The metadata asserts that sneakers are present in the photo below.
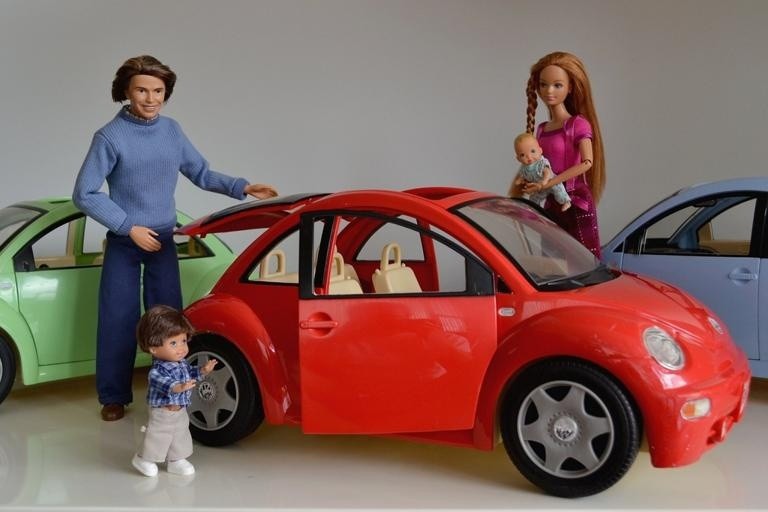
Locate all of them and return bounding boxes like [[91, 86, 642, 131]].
[[100, 403, 125, 422], [128, 453, 197, 478]]
